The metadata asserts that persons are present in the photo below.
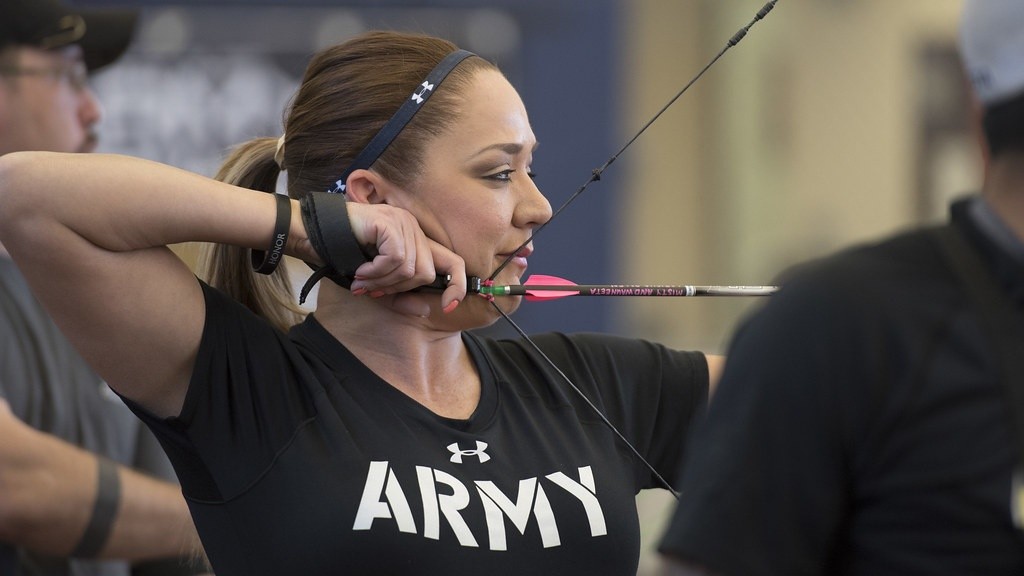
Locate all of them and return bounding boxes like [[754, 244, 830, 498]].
[[650, 1, 1024, 576], [0, 31, 722, 576], [1, 0, 219, 576]]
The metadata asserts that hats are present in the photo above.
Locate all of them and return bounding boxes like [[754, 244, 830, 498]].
[[956, 0, 1023, 106]]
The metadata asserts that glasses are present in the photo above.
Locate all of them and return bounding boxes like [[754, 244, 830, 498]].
[[2, 44, 90, 119]]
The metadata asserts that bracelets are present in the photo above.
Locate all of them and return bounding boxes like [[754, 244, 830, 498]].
[[253, 188, 292, 274], [71, 449, 123, 559]]
